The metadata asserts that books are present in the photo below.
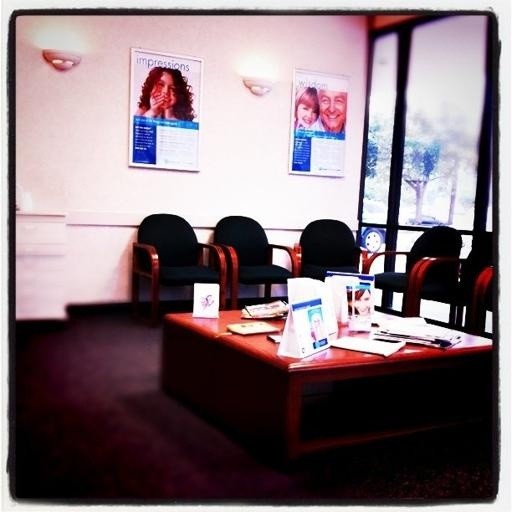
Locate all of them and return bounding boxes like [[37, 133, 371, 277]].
[[331, 336, 406, 358], [241, 300, 289, 319], [373, 324, 463, 350], [226, 321, 279, 334]]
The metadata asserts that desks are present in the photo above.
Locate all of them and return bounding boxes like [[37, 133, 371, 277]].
[[159, 300, 492, 471]]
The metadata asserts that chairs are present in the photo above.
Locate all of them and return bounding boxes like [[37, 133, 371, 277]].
[[409, 230, 493, 337], [291, 218, 369, 283], [211, 215, 297, 311], [363, 221, 457, 328], [129, 211, 228, 323]]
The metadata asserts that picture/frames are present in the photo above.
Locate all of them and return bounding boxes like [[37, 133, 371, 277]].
[[128, 47, 202, 174], [288, 66, 352, 179]]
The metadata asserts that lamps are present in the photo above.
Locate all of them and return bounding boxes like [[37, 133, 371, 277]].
[[42, 47, 82, 72], [242, 74, 277, 97]]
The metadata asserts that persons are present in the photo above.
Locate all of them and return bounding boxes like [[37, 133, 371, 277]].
[[205, 294, 214, 308], [355, 288, 371, 315], [311, 312, 323, 338], [310, 89, 347, 132], [295, 86, 321, 130], [135, 67, 194, 121]]
